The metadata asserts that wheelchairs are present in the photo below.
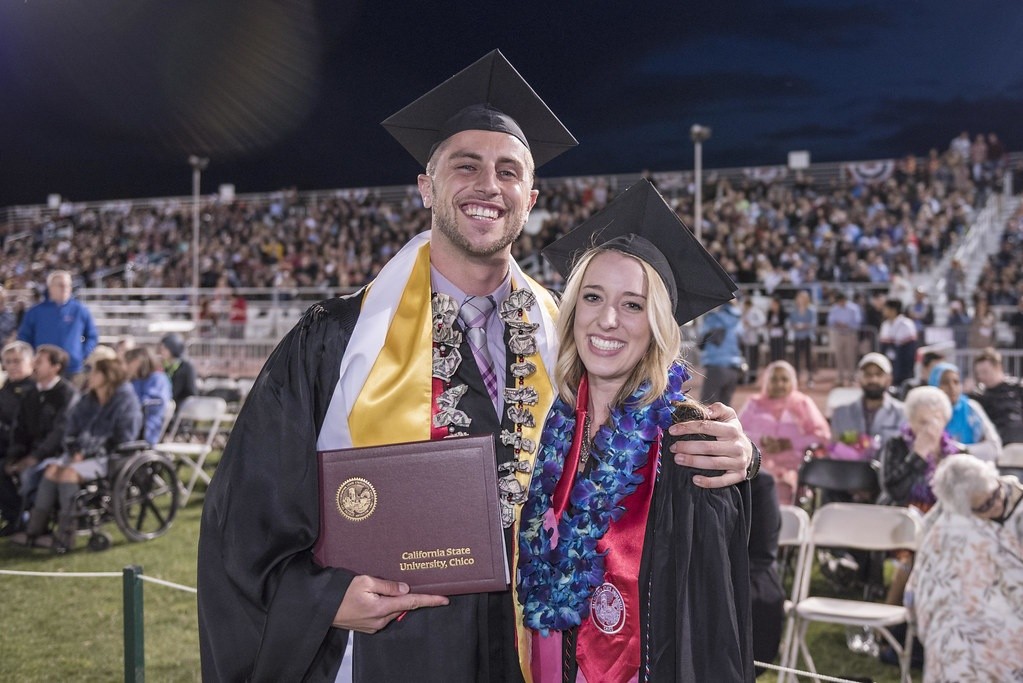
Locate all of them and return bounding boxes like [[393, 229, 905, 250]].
[[7, 440, 187, 557]]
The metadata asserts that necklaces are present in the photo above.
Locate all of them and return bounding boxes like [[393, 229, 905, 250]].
[[515, 358, 692, 638], [579, 416, 592, 463], [431, 281, 540, 529]]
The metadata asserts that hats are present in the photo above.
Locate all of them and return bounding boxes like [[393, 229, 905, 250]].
[[858, 352, 893, 377], [84, 345, 117, 371], [544, 175, 742, 324], [381, 48, 583, 169]]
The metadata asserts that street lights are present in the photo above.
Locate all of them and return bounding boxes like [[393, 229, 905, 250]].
[[691, 122, 714, 243], [188, 155, 209, 337]]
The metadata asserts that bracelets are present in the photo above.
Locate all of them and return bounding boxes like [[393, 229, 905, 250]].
[[746, 443, 761, 480]]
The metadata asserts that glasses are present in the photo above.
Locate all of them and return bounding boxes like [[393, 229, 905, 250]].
[[971, 479, 1002, 514]]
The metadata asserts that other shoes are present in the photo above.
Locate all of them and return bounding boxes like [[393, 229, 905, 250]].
[[52, 523, 76, 556], [11, 525, 47, 545]]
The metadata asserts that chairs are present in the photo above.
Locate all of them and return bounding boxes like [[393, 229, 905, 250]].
[[783, 501, 924, 683], [145, 373, 256, 509], [753, 503, 819, 683], [781, 457, 882, 600]]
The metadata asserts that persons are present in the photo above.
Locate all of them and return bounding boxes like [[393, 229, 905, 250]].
[[122, 346, 172, 446], [951, 129, 1009, 168], [13, 344, 143, 550], [0, 341, 81, 537], [228, 192, 432, 255], [515, 177, 756, 683], [15, 269, 99, 384], [511, 169, 696, 287], [0, 288, 23, 354], [702, 148, 1023, 329], [827, 294, 861, 386], [0, 208, 396, 301], [737, 347, 1023, 643], [878, 299, 918, 399], [198, 49, 759, 683], [696, 298, 746, 405], [766, 288, 815, 389], [159, 332, 197, 432]]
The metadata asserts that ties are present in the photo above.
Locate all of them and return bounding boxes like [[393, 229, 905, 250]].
[[459, 294, 500, 415]]
